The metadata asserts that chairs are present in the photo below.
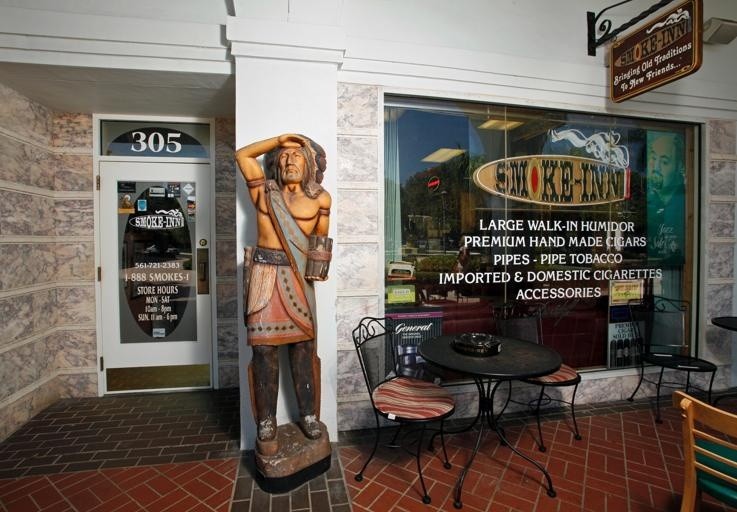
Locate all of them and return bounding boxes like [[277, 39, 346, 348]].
[[352, 313, 451, 504], [624, 291, 737, 511], [493, 297, 584, 454]]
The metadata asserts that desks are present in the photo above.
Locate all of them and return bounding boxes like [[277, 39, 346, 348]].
[[412, 331, 563, 510]]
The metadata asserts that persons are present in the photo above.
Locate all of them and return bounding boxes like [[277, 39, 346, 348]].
[[234, 134, 332, 442], [646, 137, 684, 257]]
